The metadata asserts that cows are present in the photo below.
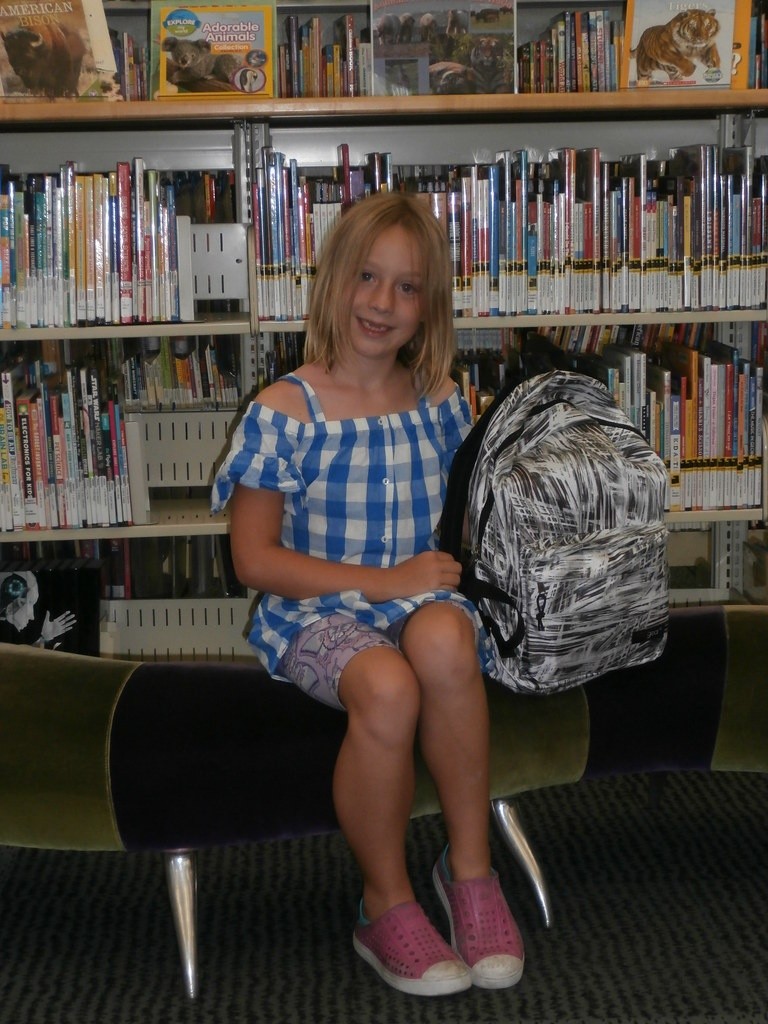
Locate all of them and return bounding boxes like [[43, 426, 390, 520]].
[[471, 6, 513, 22]]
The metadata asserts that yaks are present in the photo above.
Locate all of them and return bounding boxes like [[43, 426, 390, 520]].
[[0, 18, 89, 98]]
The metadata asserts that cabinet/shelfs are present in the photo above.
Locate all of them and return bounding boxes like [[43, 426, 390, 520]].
[[0, 0, 768, 662]]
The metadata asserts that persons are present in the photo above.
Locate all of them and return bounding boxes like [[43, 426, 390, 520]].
[[208, 192, 524, 994]]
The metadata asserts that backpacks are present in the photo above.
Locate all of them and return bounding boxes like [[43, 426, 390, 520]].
[[439, 334, 670, 693]]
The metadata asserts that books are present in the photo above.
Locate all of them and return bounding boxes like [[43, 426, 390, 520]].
[[109, 27, 151, 101], [0, 144, 768, 329], [452, 322, 768, 534], [518, 10, 625, 94], [278, 14, 371, 98], [0, 333, 308, 598], [749, 14, 768, 89]]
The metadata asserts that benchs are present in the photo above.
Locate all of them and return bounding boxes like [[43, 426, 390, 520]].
[[0, 604, 768, 998]]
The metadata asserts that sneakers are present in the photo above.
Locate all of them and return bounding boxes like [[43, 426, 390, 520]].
[[354, 901, 472, 996], [432, 842, 525, 990]]
